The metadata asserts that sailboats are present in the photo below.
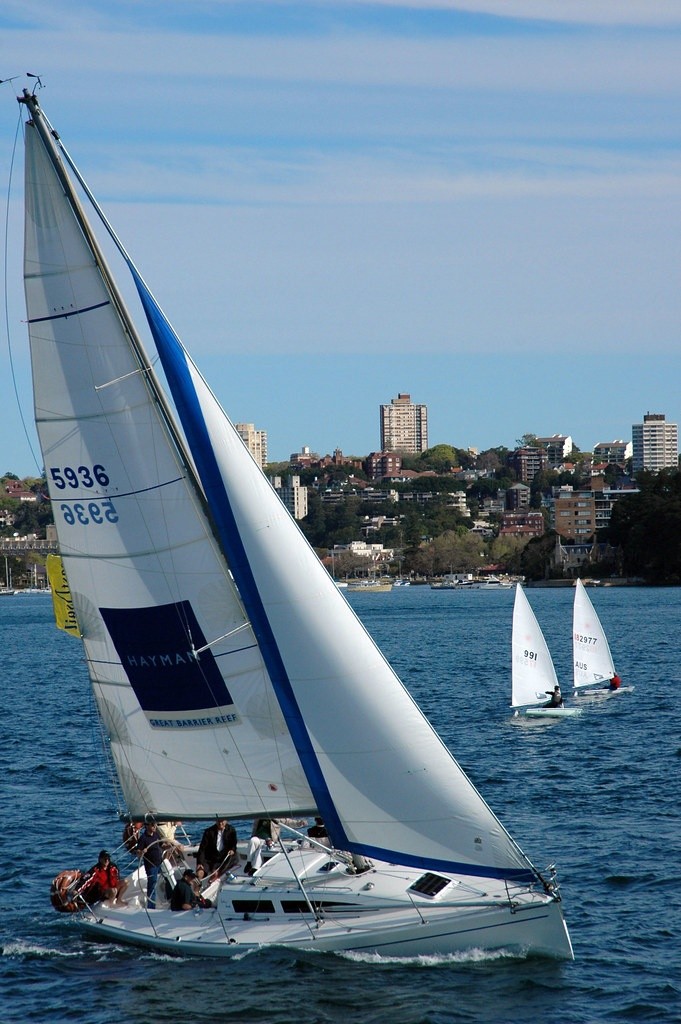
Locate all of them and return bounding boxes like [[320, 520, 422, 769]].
[[571, 577, 636, 696], [508, 582, 584, 718], [4, 66, 579, 961]]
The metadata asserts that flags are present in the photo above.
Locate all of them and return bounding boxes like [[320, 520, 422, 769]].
[[45, 553, 81, 639]]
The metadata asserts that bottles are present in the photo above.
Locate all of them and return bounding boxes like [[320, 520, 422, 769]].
[[194, 904, 200, 916], [226, 870, 234, 879]]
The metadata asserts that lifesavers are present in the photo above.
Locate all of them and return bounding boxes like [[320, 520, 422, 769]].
[[123, 822, 145, 856], [50, 870, 90, 914]]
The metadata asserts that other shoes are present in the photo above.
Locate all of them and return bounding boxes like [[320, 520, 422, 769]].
[[244, 862, 252, 872], [248, 868, 256, 876]]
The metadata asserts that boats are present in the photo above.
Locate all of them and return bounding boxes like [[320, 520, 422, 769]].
[[347, 576, 393, 593], [429, 563, 514, 590], [583, 578, 602, 588], [392, 579, 410, 588]]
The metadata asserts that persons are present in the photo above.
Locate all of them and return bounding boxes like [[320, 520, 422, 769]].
[[302, 817, 332, 849], [603, 672, 621, 691], [91, 850, 132, 908], [541, 686, 563, 708], [123, 817, 237, 912], [244, 817, 309, 877]]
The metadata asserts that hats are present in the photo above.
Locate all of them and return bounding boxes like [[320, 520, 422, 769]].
[[100, 850, 110, 857], [184, 869, 196, 879]]
